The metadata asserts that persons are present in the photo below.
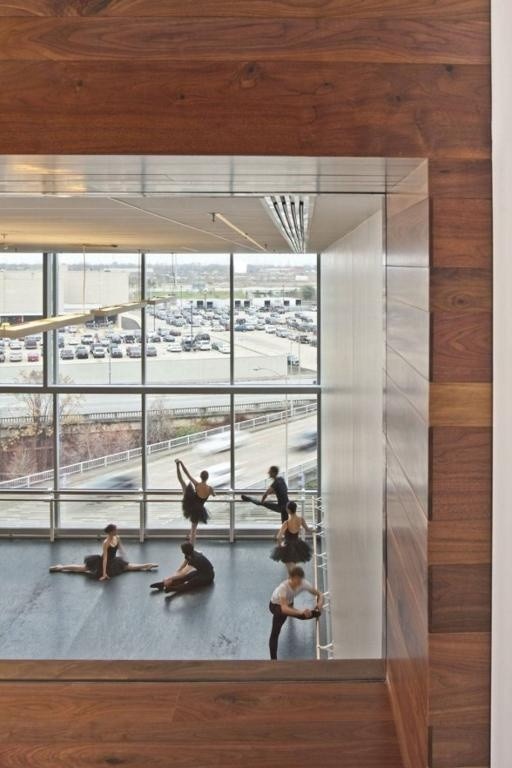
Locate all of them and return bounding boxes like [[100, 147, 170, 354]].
[[268, 567, 322, 659], [242, 466, 288, 523], [269, 501, 314, 574], [175, 457, 216, 545], [48, 524, 160, 581], [150, 542, 215, 592]]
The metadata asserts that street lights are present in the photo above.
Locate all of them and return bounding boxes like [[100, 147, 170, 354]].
[[105, 319, 117, 385], [251, 368, 288, 490], [180, 285, 183, 308]]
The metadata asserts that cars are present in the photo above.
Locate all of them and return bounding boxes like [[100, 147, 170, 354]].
[[184, 460, 249, 493], [289, 426, 318, 456], [194, 429, 251, 457], [0, 298, 317, 362], [84, 471, 142, 506]]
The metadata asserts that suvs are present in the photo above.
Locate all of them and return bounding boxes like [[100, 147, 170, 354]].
[[286, 356, 300, 366]]
